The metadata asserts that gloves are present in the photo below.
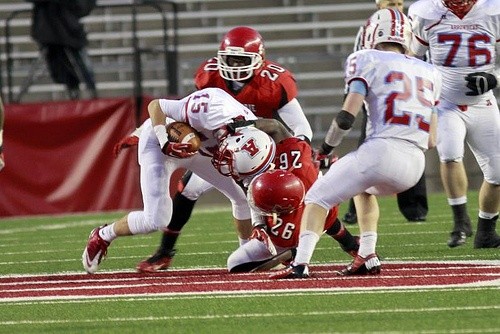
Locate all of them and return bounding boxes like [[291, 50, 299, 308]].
[[161, 140, 199, 159], [114, 135, 139, 159], [315, 151, 338, 170], [249, 224, 268, 241], [464, 72, 497, 96]]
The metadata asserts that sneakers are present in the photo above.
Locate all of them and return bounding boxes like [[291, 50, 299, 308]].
[[268, 261, 309, 279], [341, 236, 380, 261], [474, 231, 500, 249], [136, 256, 172, 273], [82, 224, 110, 276], [282, 248, 296, 267], [448, 217, 473, 247], [337, 254, 381, 277]]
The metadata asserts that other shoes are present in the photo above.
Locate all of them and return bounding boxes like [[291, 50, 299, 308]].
[[342, 212, 357, 224]]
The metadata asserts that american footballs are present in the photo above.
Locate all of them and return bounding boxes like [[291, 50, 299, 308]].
[[165, 121, 201, 153]]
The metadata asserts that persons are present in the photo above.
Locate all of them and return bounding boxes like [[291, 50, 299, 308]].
[[23, 0, 100, 100], [406, 0, 500, 249], [81, 0, 360, 273], [343, 0, 429, 224], [268, 6, 443, 278]]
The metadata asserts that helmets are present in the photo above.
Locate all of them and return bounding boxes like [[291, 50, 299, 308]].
[[211, 124, 276, 179], [218, 26, 264, 81], [360, 8, 413, 53], [247, 169, 305, 216]]
